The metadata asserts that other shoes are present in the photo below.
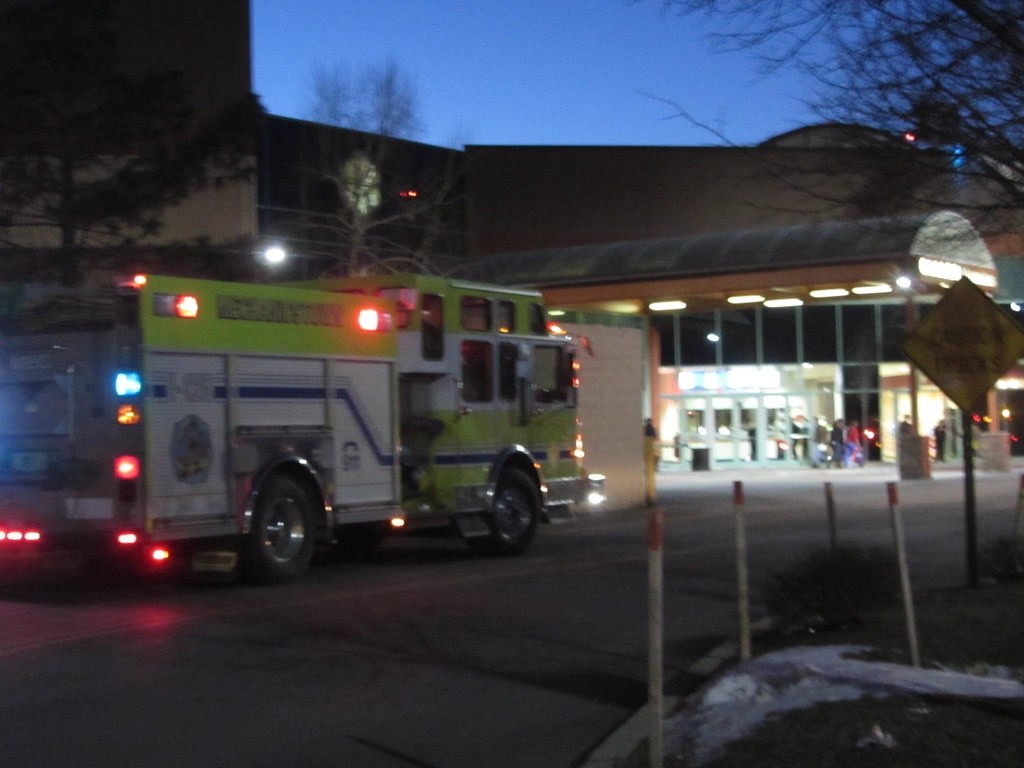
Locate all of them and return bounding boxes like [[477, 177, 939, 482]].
[[834, 461, 843, 468], [936, 456, 945, 461]]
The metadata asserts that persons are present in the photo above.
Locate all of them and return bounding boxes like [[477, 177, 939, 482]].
[[793, 415, 859, 470], [900, 415, 912, 436], [749, 424, 755, 460], [935, 421, 945, 460], [643, 418, 656, 438]]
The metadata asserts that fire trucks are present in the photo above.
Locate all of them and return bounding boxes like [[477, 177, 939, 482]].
[[0, 273, 594, 583]]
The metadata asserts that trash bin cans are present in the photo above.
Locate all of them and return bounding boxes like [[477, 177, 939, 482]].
[[689, 447, 710, 471]]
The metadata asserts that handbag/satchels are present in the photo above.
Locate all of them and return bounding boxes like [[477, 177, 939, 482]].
[[850, 448, 866, 466]]
